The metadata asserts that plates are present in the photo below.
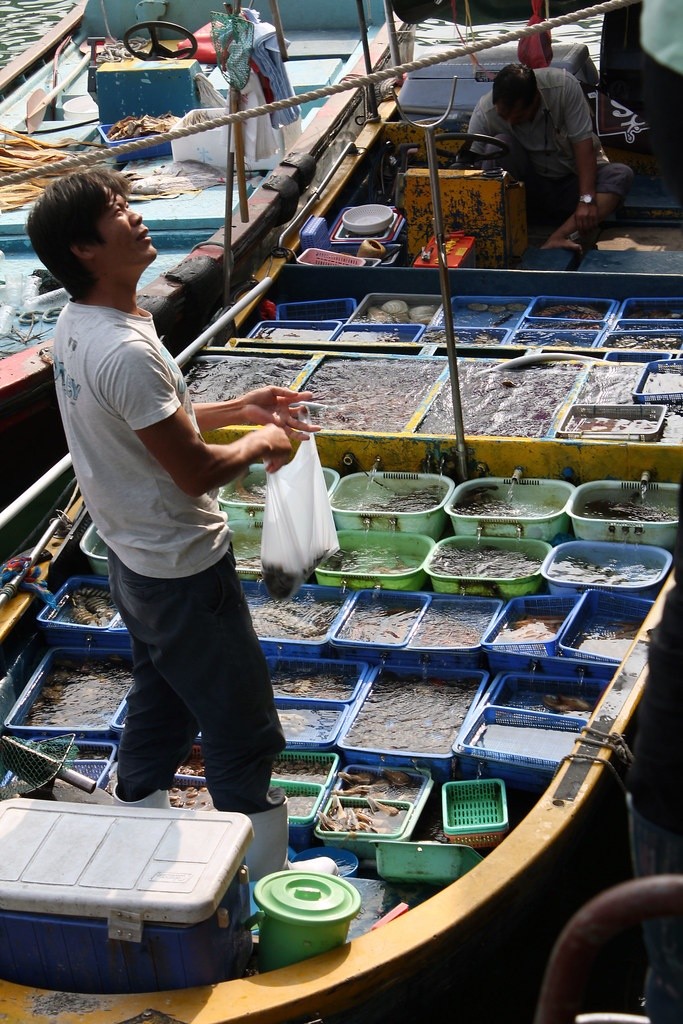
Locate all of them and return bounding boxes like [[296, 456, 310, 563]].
[[290, 847, 359, 879]]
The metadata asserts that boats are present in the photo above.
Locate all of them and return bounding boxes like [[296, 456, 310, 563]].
[[1, 1, 682, 1024], [0, 1, 408, 451]]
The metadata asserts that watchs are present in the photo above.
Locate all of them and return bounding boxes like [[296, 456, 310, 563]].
[[577, 195, 597, 206]]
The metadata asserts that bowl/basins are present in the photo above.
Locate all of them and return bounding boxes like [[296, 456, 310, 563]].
[[341, 204, 394, 235]]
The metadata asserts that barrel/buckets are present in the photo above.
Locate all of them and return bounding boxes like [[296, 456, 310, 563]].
[[245, 870, 361, 970], [62, 95, 101, 122]]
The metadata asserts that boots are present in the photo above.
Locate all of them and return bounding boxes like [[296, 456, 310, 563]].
[[113, 782, 172, 810], [245, 795, 340, 881]]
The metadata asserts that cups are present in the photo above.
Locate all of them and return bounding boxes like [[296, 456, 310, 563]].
[[354, 239, 387, 260]]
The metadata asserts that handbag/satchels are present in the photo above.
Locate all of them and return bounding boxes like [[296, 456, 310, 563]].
[[259, 405, 342, 601]]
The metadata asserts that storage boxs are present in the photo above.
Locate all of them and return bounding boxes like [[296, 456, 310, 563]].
[[0, 201, 683, 997]]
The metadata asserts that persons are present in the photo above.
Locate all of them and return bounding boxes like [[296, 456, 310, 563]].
[[28, 165, 346, 887], [466, 63, 636, 257]]
